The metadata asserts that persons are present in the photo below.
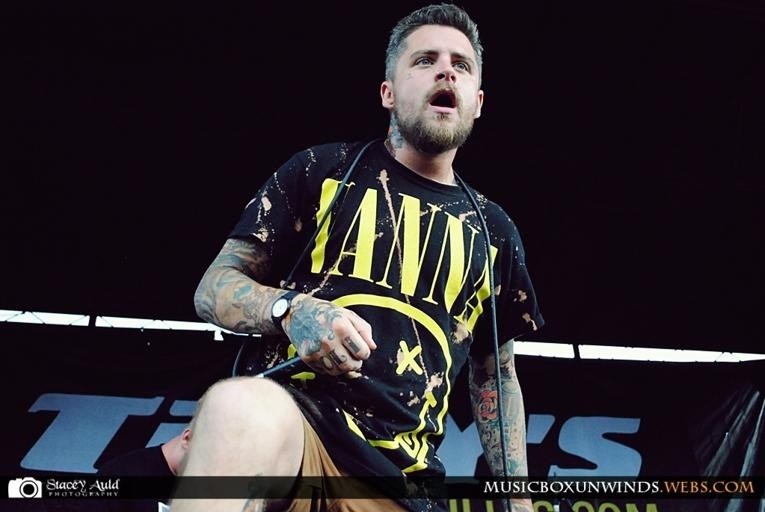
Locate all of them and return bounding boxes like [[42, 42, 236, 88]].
[[91, 413, 203, 512], [170, 0, 548, 512]]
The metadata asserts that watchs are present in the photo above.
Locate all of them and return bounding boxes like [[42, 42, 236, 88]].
[[269, 288, 303, 336]]
